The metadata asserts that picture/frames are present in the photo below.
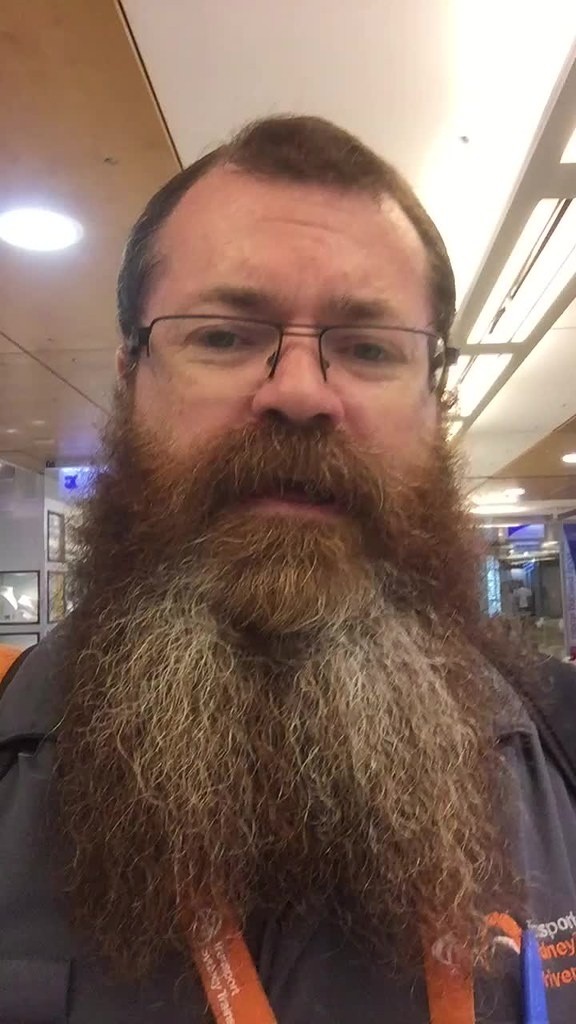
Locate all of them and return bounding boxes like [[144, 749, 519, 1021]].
[[46, 570, 66, 623], [1, 570, 41, 626], [0, 632, 40, 650], [47, 511, 66, 563]]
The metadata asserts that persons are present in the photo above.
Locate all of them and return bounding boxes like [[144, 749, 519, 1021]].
[[0, 117, 576, 1024]]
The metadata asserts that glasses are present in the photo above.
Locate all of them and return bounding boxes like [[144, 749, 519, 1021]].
[[128, 316, 461, 413]]
